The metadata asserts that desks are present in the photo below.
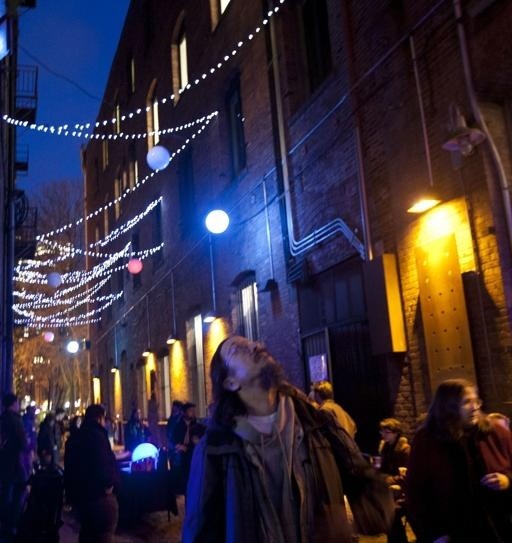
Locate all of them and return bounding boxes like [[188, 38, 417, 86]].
[[118, 473, 185, 522]]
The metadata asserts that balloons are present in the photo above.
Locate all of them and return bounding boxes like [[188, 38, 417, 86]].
[[146, 145, 170, 171], [127, 258, 143, 274], [46, 271, 60, 288]]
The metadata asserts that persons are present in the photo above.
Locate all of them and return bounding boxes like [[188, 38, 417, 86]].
[[1, 336, 511, 543]]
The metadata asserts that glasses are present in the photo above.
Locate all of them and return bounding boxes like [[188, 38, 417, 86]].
[[379, 430, 392, 435], [460, 397, 483, 410]]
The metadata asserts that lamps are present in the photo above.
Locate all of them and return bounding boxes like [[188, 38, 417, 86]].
[[439, 103, 486, 171], [256, 278, 274, 293], [91, 313, 214, 384]]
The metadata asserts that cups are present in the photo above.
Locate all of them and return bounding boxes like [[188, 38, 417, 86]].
[[398, 467, 408, 477], [373, 454, 382, 469]]
